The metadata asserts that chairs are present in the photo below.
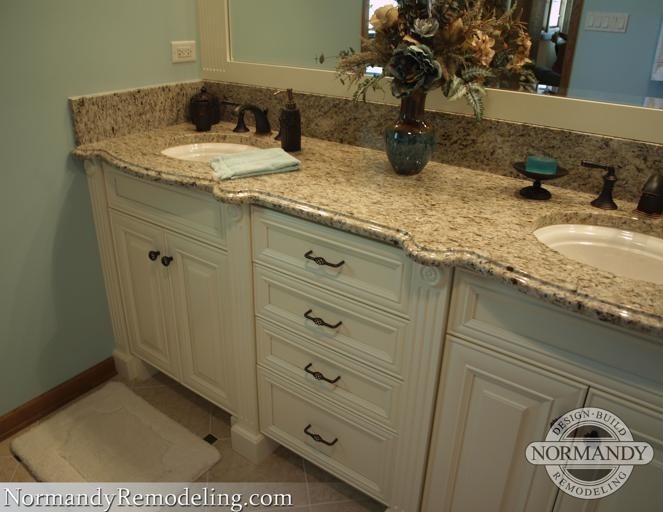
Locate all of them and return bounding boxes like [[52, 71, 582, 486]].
[[532, 30, 567, 87]]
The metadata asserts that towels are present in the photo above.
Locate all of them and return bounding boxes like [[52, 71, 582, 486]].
[[209, 147, 301, 180]]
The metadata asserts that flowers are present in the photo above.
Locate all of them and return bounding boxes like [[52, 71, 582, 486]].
[[314, 0, 510, 129], [484, 2, 537, 94]]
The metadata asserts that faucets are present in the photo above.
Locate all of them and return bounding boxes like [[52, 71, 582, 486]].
[[233, 102, 271, 135], [636, 172, 663, 217]]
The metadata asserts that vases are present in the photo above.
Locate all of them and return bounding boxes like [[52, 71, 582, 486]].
[[384, 91, 433, 176]]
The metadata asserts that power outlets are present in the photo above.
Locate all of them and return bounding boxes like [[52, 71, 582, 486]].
[[171, 40, 195, 63]]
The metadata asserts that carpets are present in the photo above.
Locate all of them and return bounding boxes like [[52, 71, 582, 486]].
[[10, 379, 223, 482]]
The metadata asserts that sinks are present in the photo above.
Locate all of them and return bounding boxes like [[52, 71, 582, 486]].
[[530, 210, 663, 286], [159, 133, 274, 163]]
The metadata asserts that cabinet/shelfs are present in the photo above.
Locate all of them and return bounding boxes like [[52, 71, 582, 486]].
[[246, 201, 454, 512], [418, 266, 663, 512], [83, 157, 261, 464]]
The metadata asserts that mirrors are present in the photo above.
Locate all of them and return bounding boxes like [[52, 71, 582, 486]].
[[194, 1, 663, 144]]
